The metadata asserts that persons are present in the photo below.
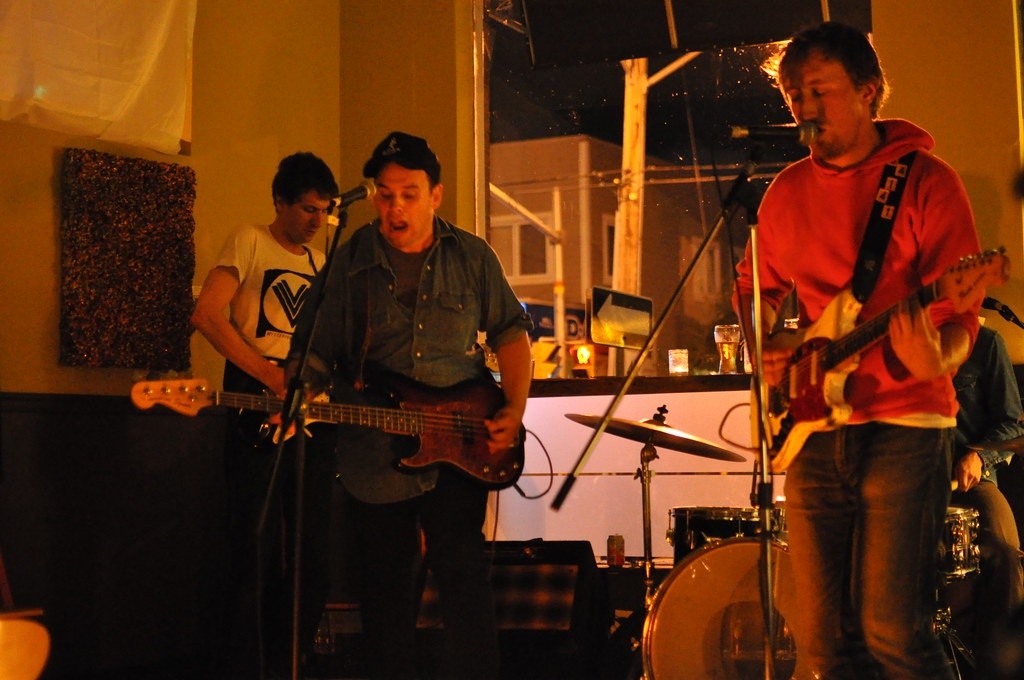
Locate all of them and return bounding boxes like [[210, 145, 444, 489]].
[[283, 132, 530, 680], [193, 149, 338, 680], [948, 326, 1024, 611], [729, 21, 986, 680]]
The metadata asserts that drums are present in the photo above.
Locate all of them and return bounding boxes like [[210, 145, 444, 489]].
[[642, 536, 797, 680], [671, 506, 784, 568], [938, 505, 983, 580]]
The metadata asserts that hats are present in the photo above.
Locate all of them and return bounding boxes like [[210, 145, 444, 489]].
[[363, 131, 440, 178]]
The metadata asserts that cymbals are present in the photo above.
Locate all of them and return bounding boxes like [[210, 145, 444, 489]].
[[564, 412, 749, 464], [967, 434, 1024, 454]]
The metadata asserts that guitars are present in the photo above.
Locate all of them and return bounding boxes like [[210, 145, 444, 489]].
[[764, 245, 1014, 476], [270, 342, 501, 445], [127, 368, 528, 491]]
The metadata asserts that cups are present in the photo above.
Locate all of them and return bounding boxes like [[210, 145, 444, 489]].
[[715, 324, 740, 375]]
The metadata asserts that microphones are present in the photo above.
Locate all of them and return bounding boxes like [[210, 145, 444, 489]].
[[328, 179, 377, 212], [724, 121, 818, 144]]
